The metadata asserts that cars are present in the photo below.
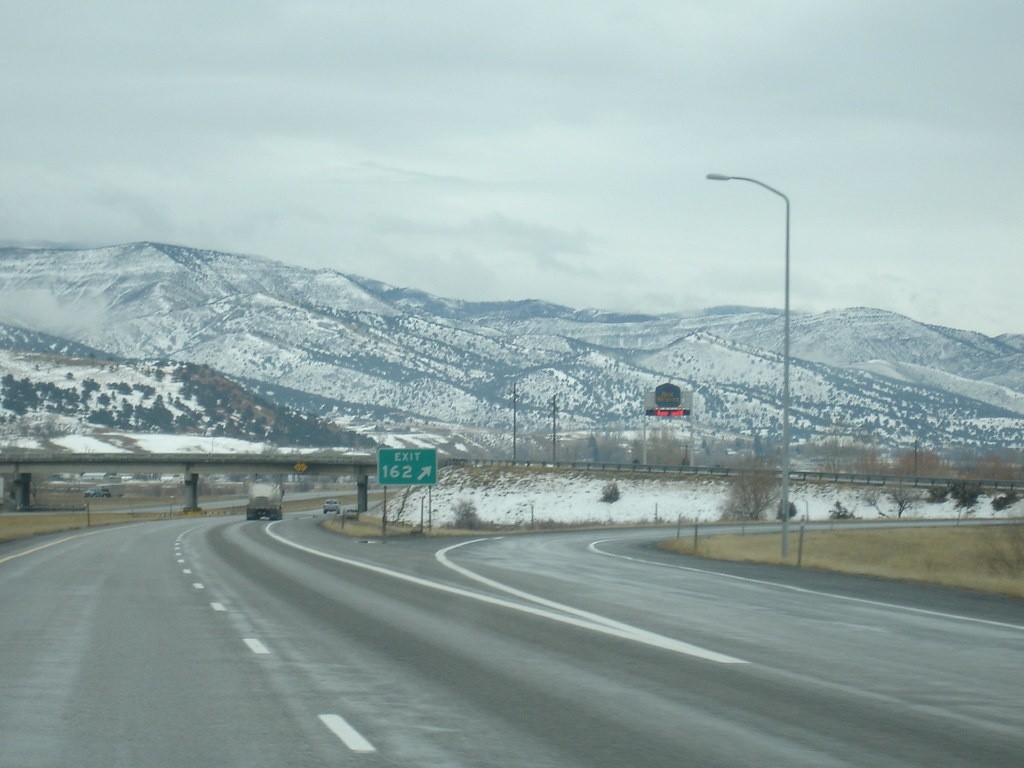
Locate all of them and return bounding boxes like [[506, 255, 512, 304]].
[[323, 499, 341, 514], [83, 486, 111, 499]]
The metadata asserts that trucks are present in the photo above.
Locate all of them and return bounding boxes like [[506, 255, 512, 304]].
[[245, 474, 285, 521]]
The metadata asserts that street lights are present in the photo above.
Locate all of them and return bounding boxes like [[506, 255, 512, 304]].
[[707, 172, 791, 558]]
